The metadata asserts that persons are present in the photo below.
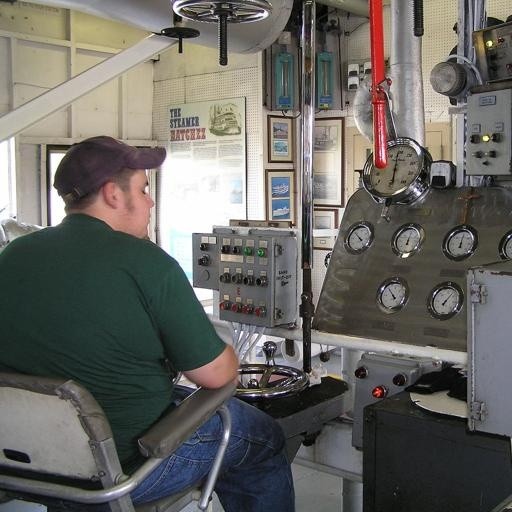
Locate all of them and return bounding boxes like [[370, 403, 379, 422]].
[[0, 136, 295, 510]]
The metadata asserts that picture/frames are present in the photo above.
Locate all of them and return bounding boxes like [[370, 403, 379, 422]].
[[267, 115, 294, 162], [313, 117, 345, 208], [312, 209, 339, 230], [266, 168, 297, 223]]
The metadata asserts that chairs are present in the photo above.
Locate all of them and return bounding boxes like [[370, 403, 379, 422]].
[[0, 370, 241, 512]]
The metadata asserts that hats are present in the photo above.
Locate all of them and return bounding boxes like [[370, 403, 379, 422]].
[[53, 137, 166, 204]]
